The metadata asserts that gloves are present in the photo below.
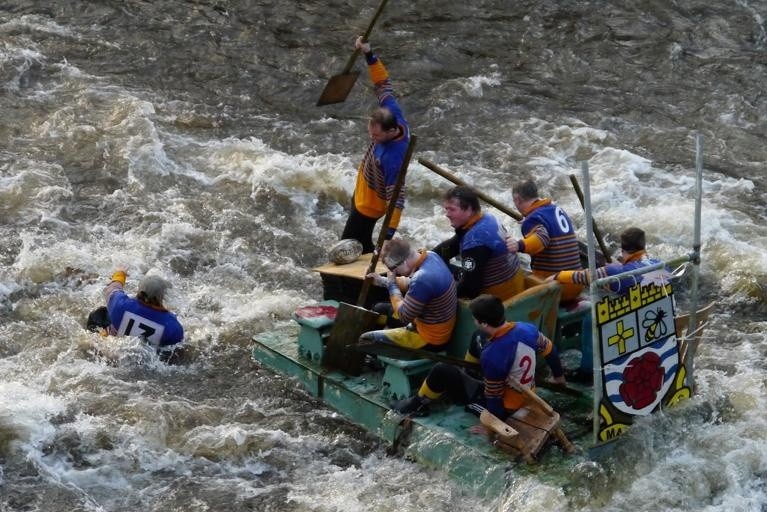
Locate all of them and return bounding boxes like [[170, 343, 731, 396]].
[[362, 267, 381, 286], [387, 271, 397, 290]]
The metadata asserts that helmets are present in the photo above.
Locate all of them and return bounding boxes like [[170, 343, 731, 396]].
[[327, 238, 364, 265]]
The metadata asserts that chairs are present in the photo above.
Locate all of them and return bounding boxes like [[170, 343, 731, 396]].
[[676, 301, 714, 366], [448, 274, 565, 363], [479, 375, 575, 465]]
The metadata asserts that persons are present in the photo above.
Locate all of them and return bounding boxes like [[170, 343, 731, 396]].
[[88, 266, 183, 347], [389, 294, 566, 422], [545, 228, 672, 384], [341, 35, 412, 261], [506, 179, 586, 305], [430, 184, 526, 303], [358, 238, 457, 372]]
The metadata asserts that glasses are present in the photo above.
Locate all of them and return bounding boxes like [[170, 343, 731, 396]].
[[388, 260, 403, 272]]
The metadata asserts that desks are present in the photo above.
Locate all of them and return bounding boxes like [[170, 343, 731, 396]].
[[310, 252, 390, 306]]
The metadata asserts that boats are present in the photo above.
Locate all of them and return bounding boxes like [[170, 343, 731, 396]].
[[248, 131, 734, 512]]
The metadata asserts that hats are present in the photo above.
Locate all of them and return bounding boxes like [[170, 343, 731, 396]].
[[137, 275, 172, 299]]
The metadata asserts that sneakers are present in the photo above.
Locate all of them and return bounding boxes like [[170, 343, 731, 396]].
[[389, 392, 430, 417], [560, 367, 592, 386]]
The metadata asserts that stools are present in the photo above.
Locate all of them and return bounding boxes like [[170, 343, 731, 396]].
[[558, 300, 593, 368], [293, 301, 340, 362], [378, 350, 447, 400]]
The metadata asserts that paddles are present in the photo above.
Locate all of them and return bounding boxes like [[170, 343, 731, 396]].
[[417, 155, 607, 270], [346, 340, 584, 400], [320, 134, 421, 377], [316, 0, 388, 106]]
